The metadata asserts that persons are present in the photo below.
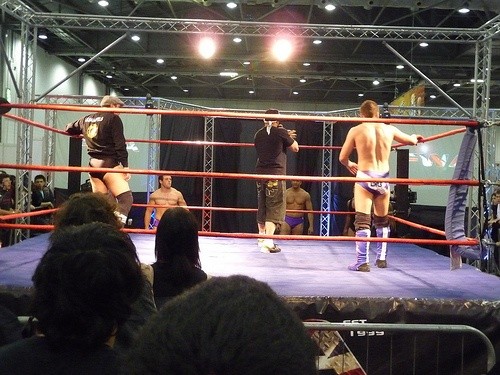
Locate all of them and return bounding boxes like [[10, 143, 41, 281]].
[[275, 179, 314, 235], [343, 196, 397, 236], [0, 171, 319, 375], [65, 95, 133, 228], [253, 108, 299, 253], [339, 99, 424, 272], [144, 175, 189, 230], [490, 189, 500, 277]]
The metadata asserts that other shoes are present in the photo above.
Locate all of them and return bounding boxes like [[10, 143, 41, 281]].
[[348, 262, 370, 271], [261, 242, 281, 253], [374, 259, 387, 268]]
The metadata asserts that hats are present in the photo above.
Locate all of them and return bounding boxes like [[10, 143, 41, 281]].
[[100, 96, 125, 107]]
